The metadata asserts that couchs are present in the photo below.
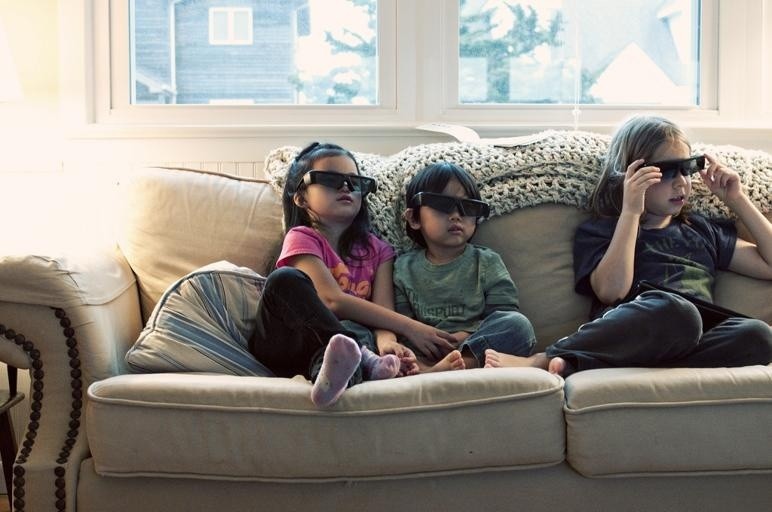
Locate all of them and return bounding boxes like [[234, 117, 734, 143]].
[[0, 134, 771, 510]]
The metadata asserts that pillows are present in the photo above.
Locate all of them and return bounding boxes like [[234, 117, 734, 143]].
[[125, 258, 276, 375]]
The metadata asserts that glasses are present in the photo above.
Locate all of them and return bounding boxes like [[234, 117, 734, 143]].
[[295, 170, 378, 195], [639, 155, 706, 180], [409, 193, 491, 219]]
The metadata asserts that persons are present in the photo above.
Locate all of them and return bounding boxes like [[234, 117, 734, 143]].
[[249, 142, 459, 405], [394, 162, 537, 374], [483, 114, 772, 381]]
